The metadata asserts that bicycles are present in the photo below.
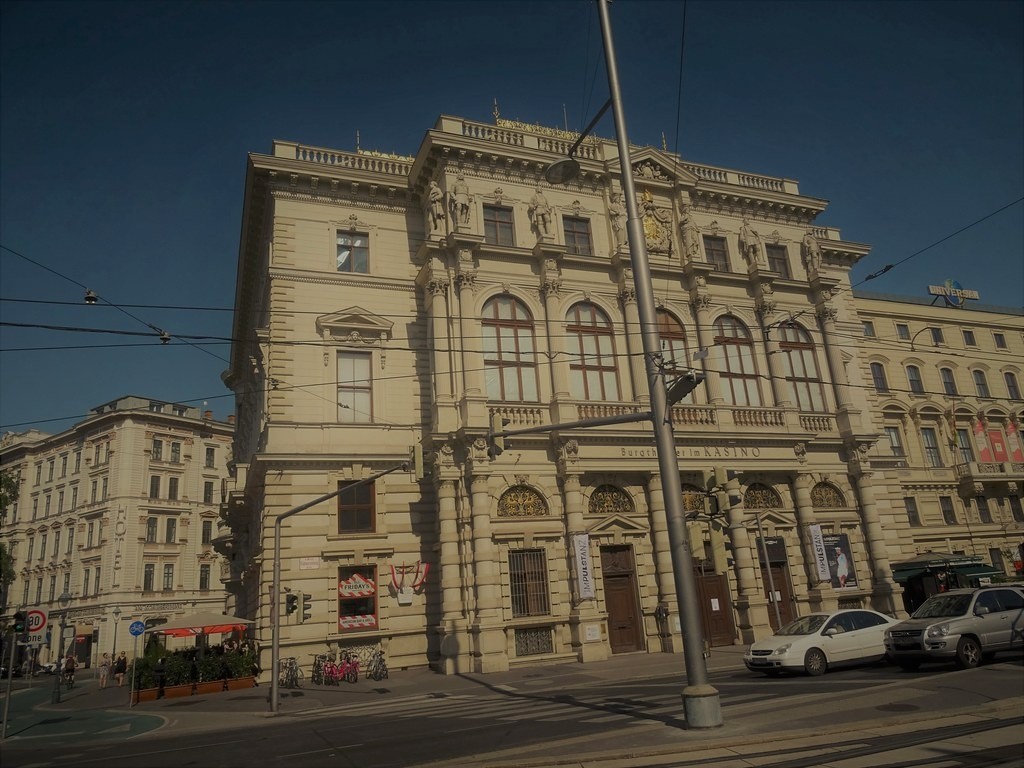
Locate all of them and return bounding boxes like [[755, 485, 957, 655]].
[[307, 645, 389, 686], [278, 656, 305, 689]]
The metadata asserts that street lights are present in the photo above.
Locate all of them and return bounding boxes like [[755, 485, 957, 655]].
[[51, 588, 73, 702], [545, 97, 723, 731]]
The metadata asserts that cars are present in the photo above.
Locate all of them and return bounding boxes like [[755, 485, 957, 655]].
[[743, 608, 905, 676], [0, 656, 22, 678], [45, 662, 66, 674]]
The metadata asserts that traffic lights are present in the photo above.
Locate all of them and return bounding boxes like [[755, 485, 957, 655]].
[[297, 592, 312, 625], [12, 611, 29, 635], [286, 593, 298, 615]]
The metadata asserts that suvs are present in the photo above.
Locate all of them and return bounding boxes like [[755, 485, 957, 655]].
[[883, 582, 1024, 669]]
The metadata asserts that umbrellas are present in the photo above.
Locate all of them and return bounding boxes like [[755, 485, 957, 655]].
[[143, 611, 254, 681]]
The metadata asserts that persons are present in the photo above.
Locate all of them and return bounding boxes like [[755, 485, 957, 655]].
[[803, 228, 822, 271], [529, 186, 554, 236], [740, 217, 763, 263], [680, 207, 699, 255], [449, 173, 472, 224], [427, 181, 445, 230], [113, 651, 128, 688], [98, 652, 112, 689], [608, 196, 628, 246], [60, 655, 78, 684], [834, 544, 849, 587]]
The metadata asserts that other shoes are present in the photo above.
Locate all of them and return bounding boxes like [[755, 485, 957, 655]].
[[114, 684, 118, 687], [117, 685, 121, 688], [102, 686, 107, 689], [98, 687, 102, 690]]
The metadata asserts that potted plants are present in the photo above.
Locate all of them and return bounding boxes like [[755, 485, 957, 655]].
[[128, 641, 255, 703]]
